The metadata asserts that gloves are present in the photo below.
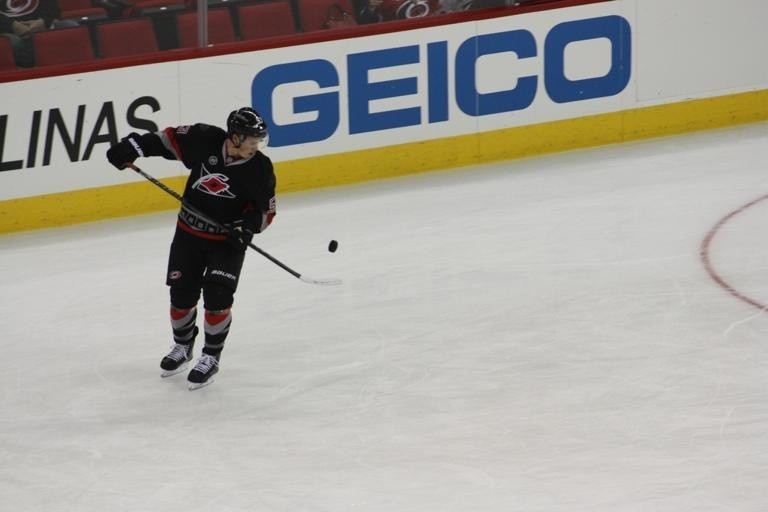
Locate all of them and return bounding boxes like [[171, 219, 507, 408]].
[[229, 221, 255, 253], [106, 133, 149, 169]]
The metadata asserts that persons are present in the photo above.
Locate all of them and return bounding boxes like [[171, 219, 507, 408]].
[[0, 0, 60, 68], [106, 107, 276, 383], [322, 0, 520, 28]]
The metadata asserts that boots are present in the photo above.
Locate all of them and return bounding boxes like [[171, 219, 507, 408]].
[[187, 339, 224, 383], [161, 326, 198, 370]]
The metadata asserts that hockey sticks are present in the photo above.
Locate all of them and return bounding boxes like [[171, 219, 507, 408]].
[[129, 163, 338, 284]]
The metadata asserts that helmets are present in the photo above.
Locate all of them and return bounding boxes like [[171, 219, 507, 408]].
[[227, 108, 269, 150]]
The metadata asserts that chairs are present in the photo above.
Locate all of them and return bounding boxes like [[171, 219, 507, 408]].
[[0, 0, 355, 72]]
